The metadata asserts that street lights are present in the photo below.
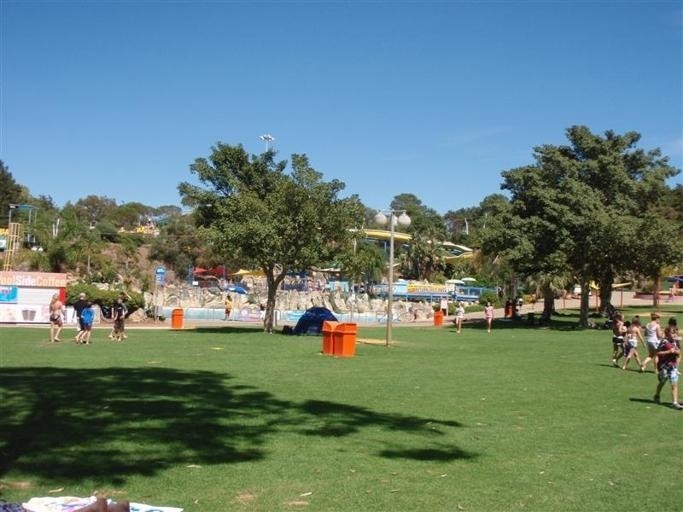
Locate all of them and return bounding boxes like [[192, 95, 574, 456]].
[[373, 207, 412, 347], [259, 133, 276, 152]]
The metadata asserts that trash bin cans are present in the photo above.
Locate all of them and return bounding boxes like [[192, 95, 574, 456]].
[[171, 308, 184, 329], [434, 311, 443, 327], [321, 320, 357, 357]]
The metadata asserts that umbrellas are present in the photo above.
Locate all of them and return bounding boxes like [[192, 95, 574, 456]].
[[225, 285, 248, 296], [461, 276, 476, 285], [193, 265, 226, 274], [232, 267, 267, 277], [446, 279, 463, 292]]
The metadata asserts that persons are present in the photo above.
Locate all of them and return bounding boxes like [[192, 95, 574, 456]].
[[453, 301, 464, 332], [483, 300, 494, 333], [47, 291, 132, 344], [222, 294, 232, 320], [0, 492, 130, 512], [609, 309, 683, 413], [502, 296, 550, 327]]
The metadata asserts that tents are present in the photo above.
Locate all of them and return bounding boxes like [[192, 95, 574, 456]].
[[292, 305, 338, 335]]
